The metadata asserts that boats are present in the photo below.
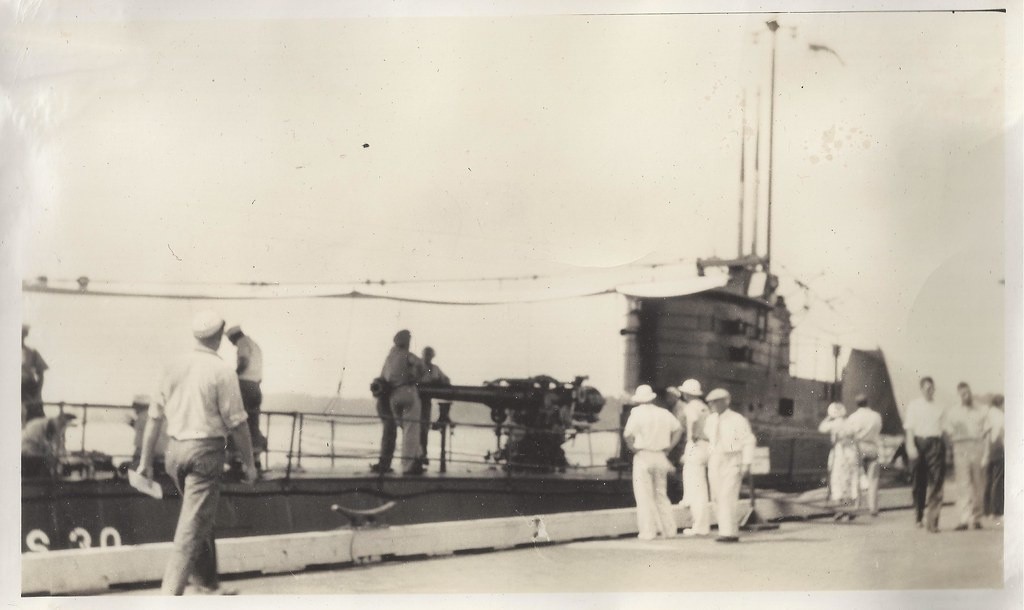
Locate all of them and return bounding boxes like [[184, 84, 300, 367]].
[[605, 21, 923, 493]]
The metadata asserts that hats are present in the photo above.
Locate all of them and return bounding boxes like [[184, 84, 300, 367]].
[[226, 325, 240, 338], [134, 395, 150, 406], [678, 379, 703, 396], [192, 314, 223, 338], [828, 402, 846, 418], [704, 388, 728, 402], [631, 384, 656, 402]]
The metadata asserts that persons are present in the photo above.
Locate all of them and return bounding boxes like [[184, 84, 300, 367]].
[[21, 321, 77, 476], [819, 394, 882, 519], [116, 396, 171, 478], [901, 377, 1005, 533], [623, 377, 758, 543], [137, 318, 258, 595], [225, 323, 267, 468], [372, 329, 453, 476]]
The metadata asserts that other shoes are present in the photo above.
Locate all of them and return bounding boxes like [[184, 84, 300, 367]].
[[973, 523, 981, 529], [717, 536, 738, 542], [955, 524, 967, 531]]
